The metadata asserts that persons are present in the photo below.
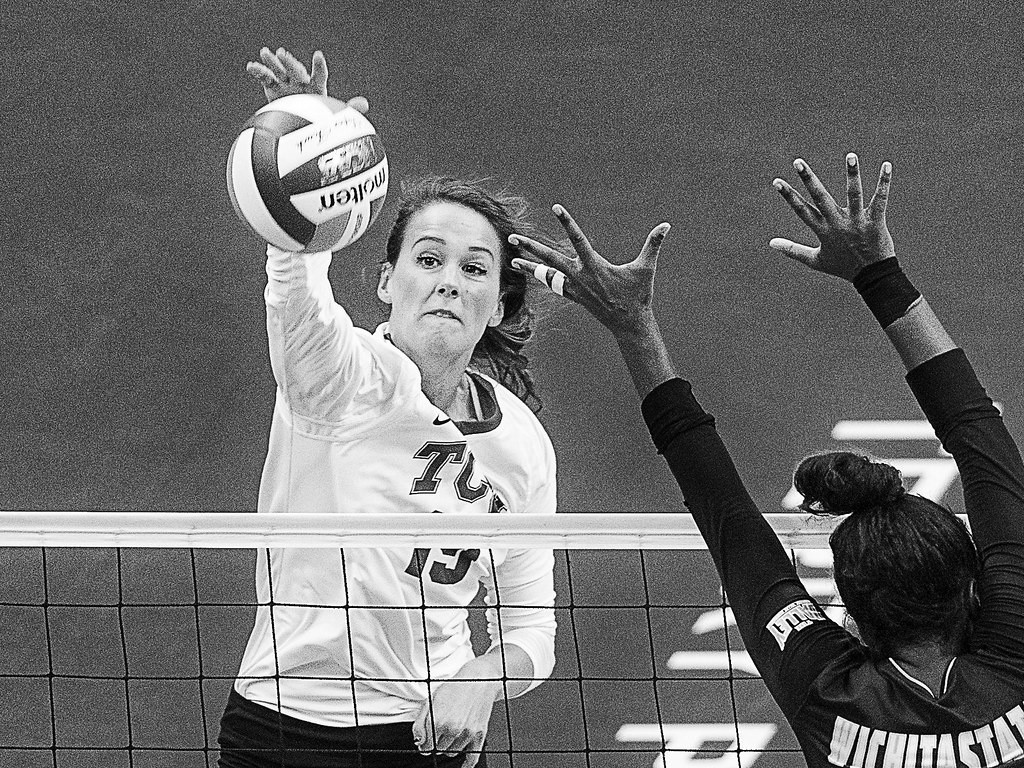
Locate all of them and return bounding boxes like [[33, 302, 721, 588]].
[[506, 152, 1024, 768], [215, 46, 560, 768]]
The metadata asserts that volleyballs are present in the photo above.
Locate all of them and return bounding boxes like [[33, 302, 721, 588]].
[[226, 93, 389, 254]]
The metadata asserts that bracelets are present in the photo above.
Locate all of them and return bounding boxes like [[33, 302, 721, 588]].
[[850, 257, 921, 327]]
